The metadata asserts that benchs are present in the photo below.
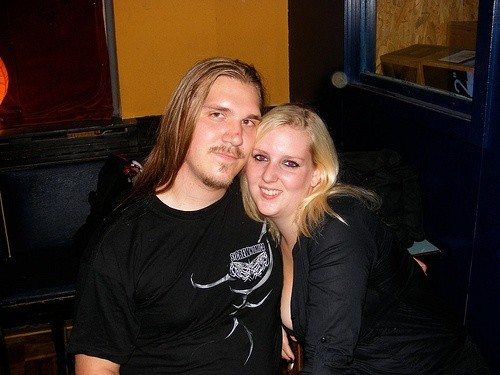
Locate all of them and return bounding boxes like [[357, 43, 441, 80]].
[[2, 103, 335, 375]]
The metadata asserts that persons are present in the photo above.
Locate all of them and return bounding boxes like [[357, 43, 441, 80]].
[[72, 56, 285, 375], [238, 104, 499, 374]]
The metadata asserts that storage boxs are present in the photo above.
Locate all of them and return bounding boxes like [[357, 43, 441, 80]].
[[381, 19, 478, 101]]
